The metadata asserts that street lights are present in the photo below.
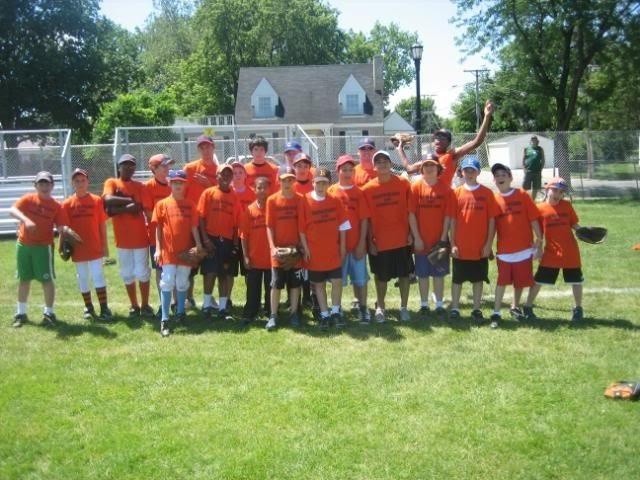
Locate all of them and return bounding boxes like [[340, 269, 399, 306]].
[[409, 36, 424, 135]]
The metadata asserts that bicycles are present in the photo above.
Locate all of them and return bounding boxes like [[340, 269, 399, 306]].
[[533, 184, 573, 207]]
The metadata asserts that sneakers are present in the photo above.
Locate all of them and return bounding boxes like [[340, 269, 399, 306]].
[[160, 320, 170, 336], [419, 306, 429, 321], [302, 295, 313, 309], [187, 298, 197, 310], [435, 307, 446, 321], [40, 313, 56, 326], [12, 314, 27, 328], [175, 309, 188, 323], [224, 299, 232, 314], [201, 305, 213, 322], [84, 306, 94, 321], [523, 303, 536, 319], [572, 306, 582, 321], [330, 313, 345, 330], [351, 300, 360, 315], [141, 305, 155, 319], [319, 316, 330, 331], [471, 310, 486, 325], [218, 310, 235, 321], [209, 296, 219, 310], [156, 305, 162, 320], [100, 307, 113, 322], [399, 309, 411, 322], [374, 307, 384, 323], [244, 313, 258, 324], [490, 314, 501, 329], [129, 305, 140, 321], [510, 305, 524, 320], [448, 311, 460, 323], [265, 313, 281, 328], [288, 309, 301, 328], [359, 309, 371, 325]]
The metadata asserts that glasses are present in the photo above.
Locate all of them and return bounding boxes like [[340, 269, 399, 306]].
[[434, 135, 446, 140], [360, 147, 374, 150]]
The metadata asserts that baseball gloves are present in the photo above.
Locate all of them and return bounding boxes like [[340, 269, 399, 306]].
[[427, 241, 450, 267], [60, 242, 71, 260], [390, 132, 415, 150], [176, 247, 208, 269], [278, 245, 304, 271], [574, 226, 607, 244]]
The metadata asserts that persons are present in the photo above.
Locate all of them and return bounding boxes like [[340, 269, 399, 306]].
[[10, 170, 74, 328], [394, 99, 494, 287], [102, 137, 607, 336], [61, 168, 116, 324]]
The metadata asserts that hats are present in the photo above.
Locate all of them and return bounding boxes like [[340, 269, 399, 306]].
[[491, 164, 510, 176], [232, 163, 247, 174], [197, 135, 214, 147], [278, 167, 296, 180], [118, 154, 136, 165], [149, 154, 175, 170], [313, 166, 331, 183], [292, 153, 311, 167], [35, 171, 54, 184], [421, 154, 439, 169], [71, 168, 87, 179], [284, 142, 302, 153], [169, 170, 187, 182], [358, 138, 376, 150], [433, 129, 451, 142], [460, 158, 480, 172], [544, 178, 569, 193], [216, 164, 233, 176], [336, 154, 355, 171], [372, 150, 390, 167]]
